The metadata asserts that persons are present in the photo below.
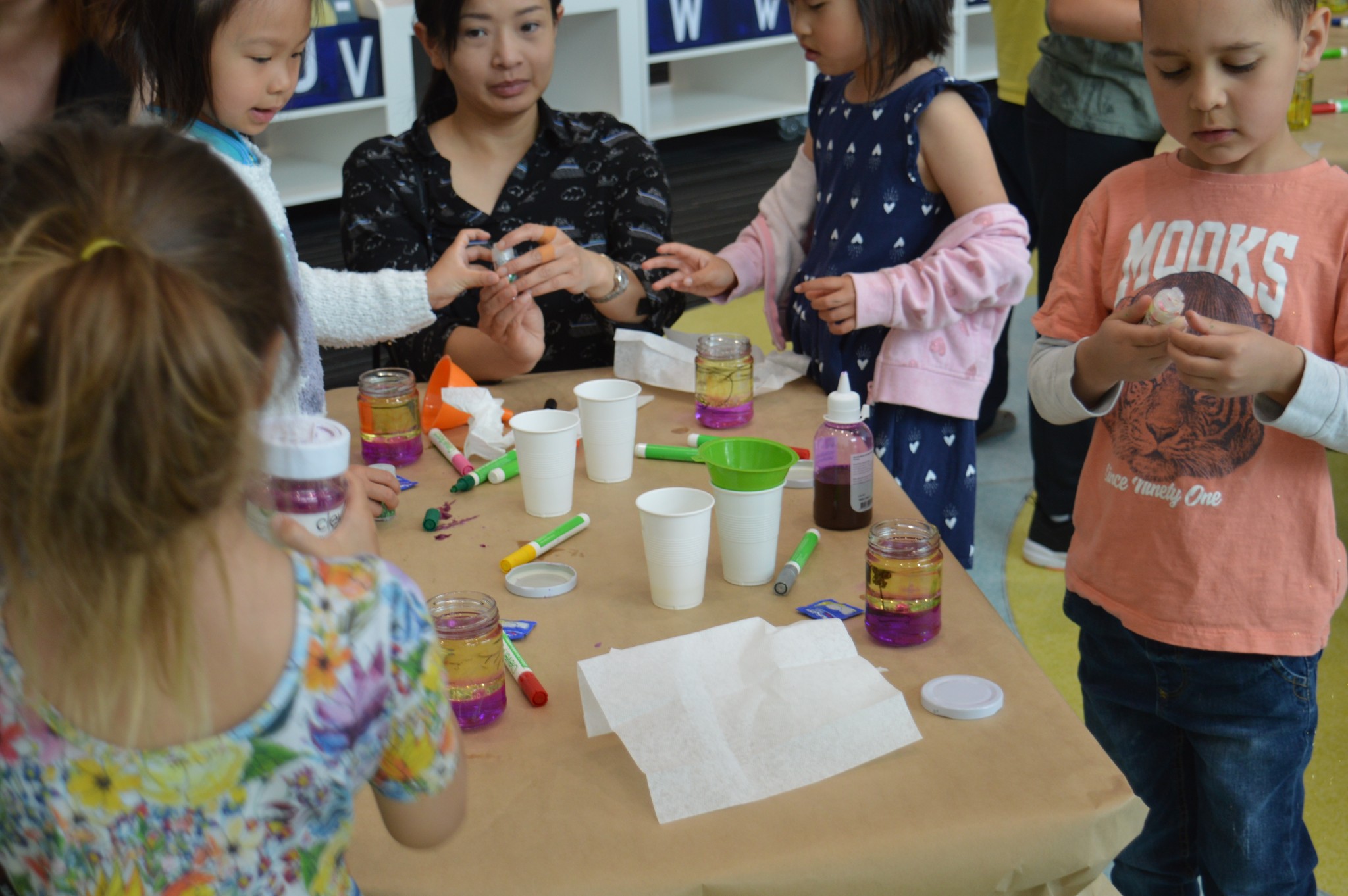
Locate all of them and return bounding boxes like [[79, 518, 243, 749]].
[[335, 0, 678, 384], [641, 0, 1032, 573], [971, 0, 1166, 570], [1019, 0, 1348, 895], [129, 0, 511, 441], [0, 0, 477, 896]]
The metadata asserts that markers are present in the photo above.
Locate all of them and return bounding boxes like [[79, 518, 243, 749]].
[[500, 511, 592, 575], [428, 427, 475, 475], [487, 440, 581, 485], [772, 527, 823, 597], [688, 431, 811, 461], [635, 442, 706, 466], [448, 448, 517, 494], [503, 631, 550, 708]]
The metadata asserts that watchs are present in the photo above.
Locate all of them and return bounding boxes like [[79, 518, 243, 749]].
[[581, 253, 629, 302]]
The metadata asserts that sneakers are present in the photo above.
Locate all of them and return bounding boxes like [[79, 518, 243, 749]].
[[1023, 495, 1075, 569]]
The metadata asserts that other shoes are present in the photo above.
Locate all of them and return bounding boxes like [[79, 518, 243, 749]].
[[974, 411, 1014, 444]]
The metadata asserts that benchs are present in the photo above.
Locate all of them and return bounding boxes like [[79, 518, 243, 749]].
[[282, 109, 810, 390]]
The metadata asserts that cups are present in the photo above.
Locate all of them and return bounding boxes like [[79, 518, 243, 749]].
[[636, 487, 715, 610], [709, 481, 786, 587], [573, 379, 642, 483], [509, 409, 579, 518]]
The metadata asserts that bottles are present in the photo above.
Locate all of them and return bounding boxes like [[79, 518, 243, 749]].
[[258, 416, 351, 593], [864, 520, 943, 647], [813, 371, 874, 532], [427, 591, 507, 732], [357, 368, 422, 468], [696, 333, 754, 429], [493, 244, 518, 298]]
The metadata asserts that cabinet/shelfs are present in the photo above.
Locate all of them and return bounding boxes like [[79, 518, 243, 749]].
[[257, 0, 998, 209]]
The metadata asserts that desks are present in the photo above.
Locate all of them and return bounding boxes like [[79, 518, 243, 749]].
[[322, 364, 1150, 896]]
